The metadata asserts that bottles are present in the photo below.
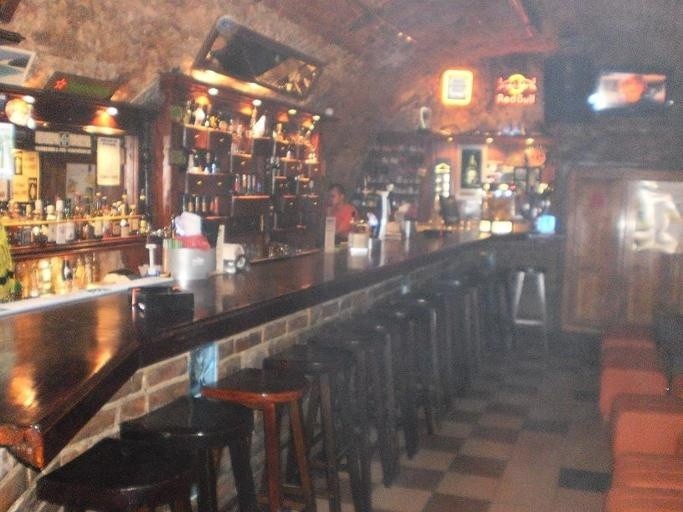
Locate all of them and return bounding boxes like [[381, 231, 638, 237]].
[[418, 106, 433, 132], [11, 254, 94, 300], [234, 173, 261, 195], [7, 187, 151, 245], [181, 100, 220, 215]]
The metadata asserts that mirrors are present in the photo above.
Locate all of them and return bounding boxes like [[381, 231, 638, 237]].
[[0, 119, 140, 221], [191, 18, 326, 102]]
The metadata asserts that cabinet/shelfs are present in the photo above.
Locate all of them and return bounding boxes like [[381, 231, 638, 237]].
[[153, 74, 332, 226]]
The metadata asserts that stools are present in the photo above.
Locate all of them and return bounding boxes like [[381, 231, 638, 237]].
[[35, 436, 218, 510], [262, 343, 364, 510], [315, 293, 442, 488], [203, 366, 317, 510], [598, 322, 683, 510], [119, 394, 257, 510]]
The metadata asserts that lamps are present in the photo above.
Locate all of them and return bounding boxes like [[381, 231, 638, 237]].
[[81, 112, 126, 137]]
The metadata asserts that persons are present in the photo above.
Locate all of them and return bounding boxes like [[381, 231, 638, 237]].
[[323, 184, 361, 242]]
